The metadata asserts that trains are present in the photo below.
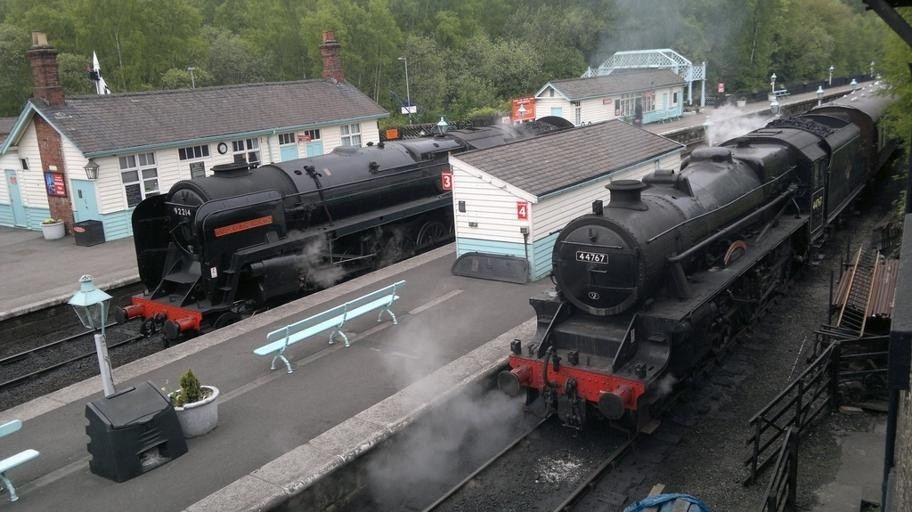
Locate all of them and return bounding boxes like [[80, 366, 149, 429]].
[[498, 83, 901, 437], [114, 113, 578, 345]]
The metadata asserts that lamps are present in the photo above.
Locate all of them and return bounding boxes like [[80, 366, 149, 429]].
[[83, 159, 99, 179]]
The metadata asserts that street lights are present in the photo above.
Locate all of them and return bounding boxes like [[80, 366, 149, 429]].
[[188, 67, 195, 89], [770, 98, 781, 118], [398, 56, 412, 126], [870, 60, 875, 78], [829, 64, 834, 85], [770, 74, 777, 93], [816, 86, 824, 105], [437, 116, 448, 135], [851, 78, 857, 92], [68, 274, 115, 396]]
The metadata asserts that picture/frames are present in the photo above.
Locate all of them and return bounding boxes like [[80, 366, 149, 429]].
[[44, 171, 67, 197]]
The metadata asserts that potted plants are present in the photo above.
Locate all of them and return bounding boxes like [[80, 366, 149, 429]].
[[165, 368, 219, 439]]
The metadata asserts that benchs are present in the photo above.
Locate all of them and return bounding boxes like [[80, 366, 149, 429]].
[[253, 280, 406, 373], [0, 420, 40, 502]]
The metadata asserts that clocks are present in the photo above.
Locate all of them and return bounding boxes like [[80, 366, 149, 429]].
[[218, 142, 227, 154]]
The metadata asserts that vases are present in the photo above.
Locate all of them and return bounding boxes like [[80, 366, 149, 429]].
[[40, 220, 65, 240]]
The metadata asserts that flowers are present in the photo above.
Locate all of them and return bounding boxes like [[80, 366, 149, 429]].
[[43, 218, 62, 224]]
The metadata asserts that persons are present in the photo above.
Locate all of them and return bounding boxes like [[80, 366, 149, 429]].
[[635, 101, 642, 127]]
